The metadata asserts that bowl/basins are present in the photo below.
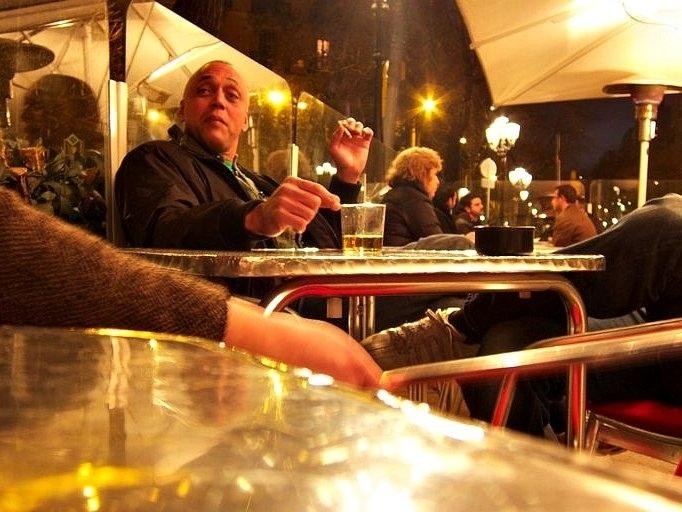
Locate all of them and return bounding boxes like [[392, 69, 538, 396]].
[[474, 225, 537, 256]]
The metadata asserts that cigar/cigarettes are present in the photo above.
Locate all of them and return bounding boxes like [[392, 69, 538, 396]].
[[337, 118, 353, 139]]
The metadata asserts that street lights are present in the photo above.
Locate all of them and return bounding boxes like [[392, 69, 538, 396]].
[[479, 116, 551, 239], [458, 136, 467, 186], [410, 95, 437, 147]]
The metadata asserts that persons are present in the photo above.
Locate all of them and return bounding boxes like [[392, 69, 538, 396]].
[[1, 186, 383, 391], [356, 192, 680, 445], [116, 60, 373, 320], [362, 146, 598, 254], [20, 74, 104, 154]]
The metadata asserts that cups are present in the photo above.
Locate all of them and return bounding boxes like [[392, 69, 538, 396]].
[[340, 202, 386, 257]]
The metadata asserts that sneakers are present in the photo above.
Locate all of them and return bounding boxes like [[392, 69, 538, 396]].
[[359, 307, 479, 417]]
